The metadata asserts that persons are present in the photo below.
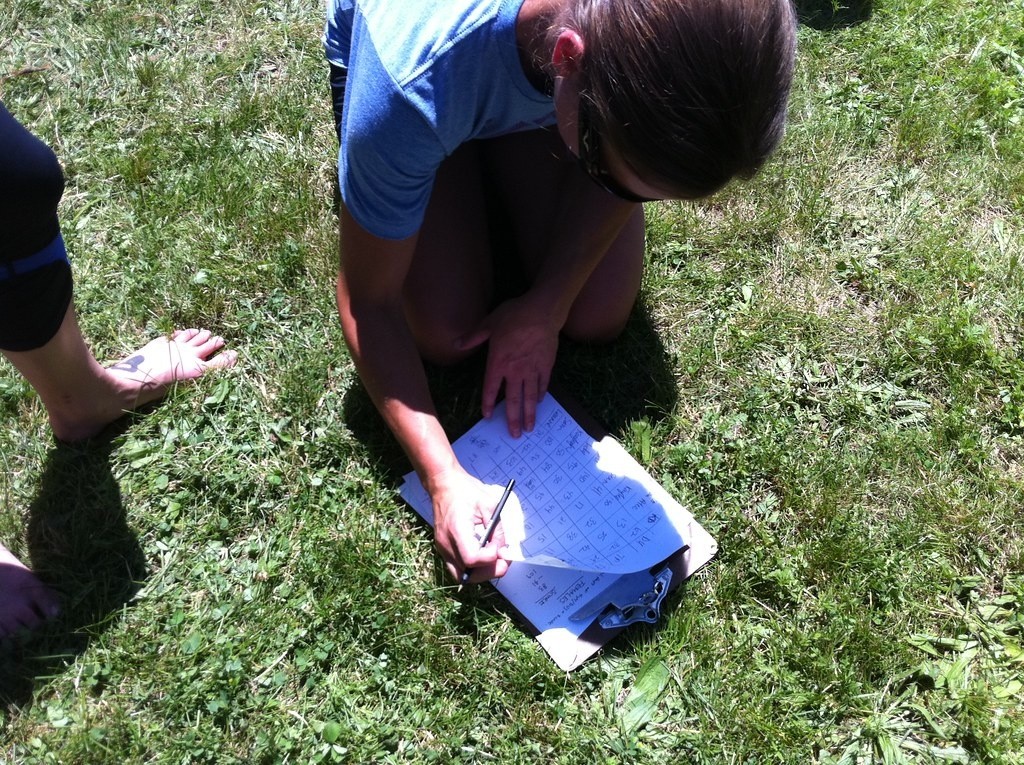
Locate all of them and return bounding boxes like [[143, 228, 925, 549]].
[[322, 0, 794, 590], [0, 101, 243, 641]]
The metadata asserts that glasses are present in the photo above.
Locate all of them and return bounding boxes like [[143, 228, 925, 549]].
[[578, 63, 658, 205]]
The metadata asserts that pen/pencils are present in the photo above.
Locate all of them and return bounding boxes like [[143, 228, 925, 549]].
[[457, 478, 516, 594]]
